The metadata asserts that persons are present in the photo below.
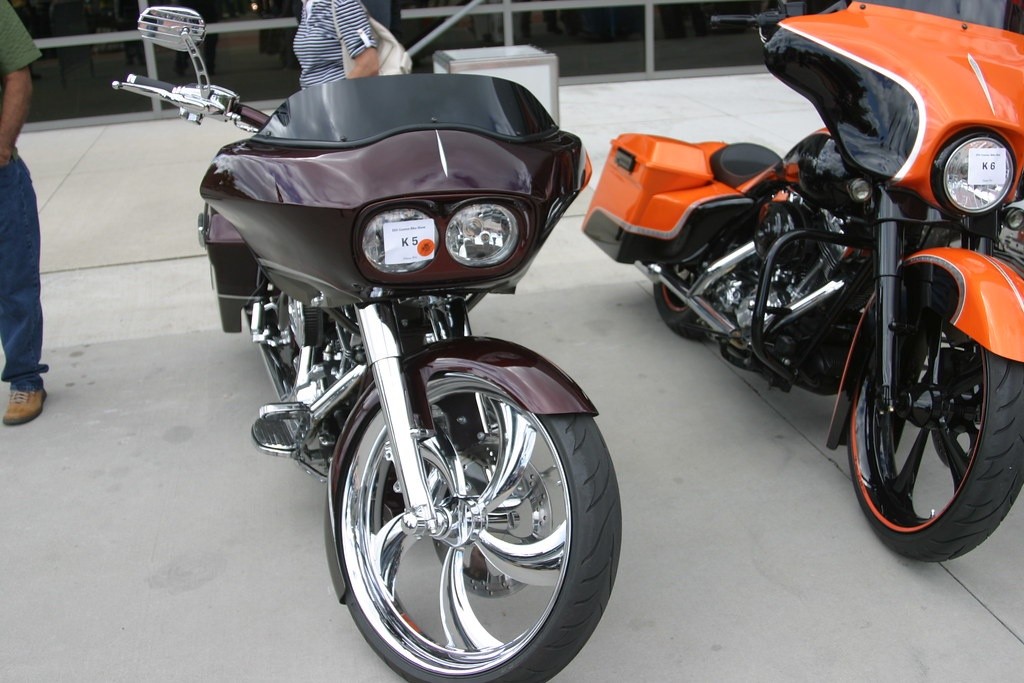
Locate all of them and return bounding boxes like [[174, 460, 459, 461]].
[[175, 0, 218, 76], [520, 10, 563, 37], [293, 0, 380, 90], [0, 0, 49, 426], [119, 9, 146, 66]]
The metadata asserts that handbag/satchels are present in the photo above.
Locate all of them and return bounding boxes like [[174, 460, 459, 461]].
[[332, 0, 413, 78]]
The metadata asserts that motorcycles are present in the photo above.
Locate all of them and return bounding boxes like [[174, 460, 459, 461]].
[[579, 0, 1023, 565], [106, 0, 622, 683]]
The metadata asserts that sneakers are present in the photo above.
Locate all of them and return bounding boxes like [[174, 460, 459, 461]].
[[3, 386, 47, 424]]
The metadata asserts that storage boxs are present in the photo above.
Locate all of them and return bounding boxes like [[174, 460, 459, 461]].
[[433, 43, 560, 131]]
